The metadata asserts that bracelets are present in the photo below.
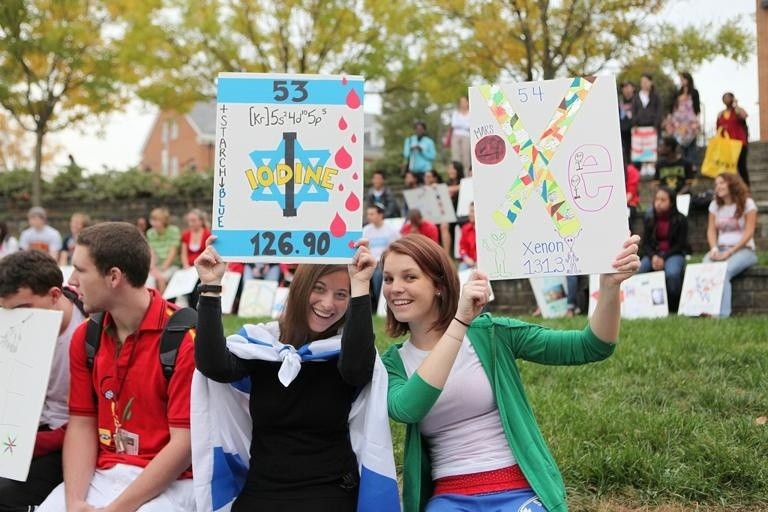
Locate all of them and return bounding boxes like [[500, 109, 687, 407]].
[[452, 315, 472, 330]]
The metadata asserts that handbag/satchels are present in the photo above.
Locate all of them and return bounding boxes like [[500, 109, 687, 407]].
[[399, 161, 415, 172], [701, 126, 744, 180]]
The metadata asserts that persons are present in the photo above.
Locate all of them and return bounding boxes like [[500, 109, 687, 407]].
[[0, 206, 213, 322], [0, 247, 90, 512], [34, 220, 197, 512], [365, 71, 759, 322], [192, 234, 378, 512], [378, 231, 644, 511]]
